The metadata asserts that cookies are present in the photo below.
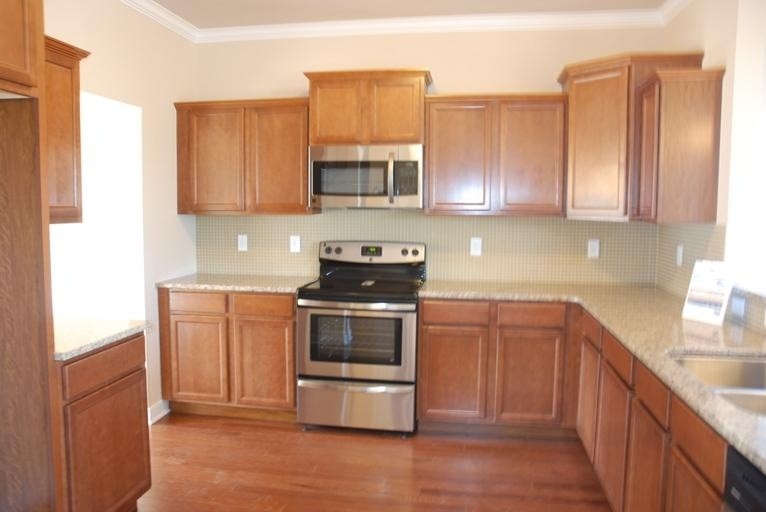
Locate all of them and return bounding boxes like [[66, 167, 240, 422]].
[[306, 143, 425, 212]]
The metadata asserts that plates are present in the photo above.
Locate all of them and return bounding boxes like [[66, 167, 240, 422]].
[[578, 306, 634, 510], [38, 44, 87, 225], [302, 68, 429, 140], [555, 57, 705, 220], [632, 73, 723, 224], [54, 328, 148, 512], [173, 97, 310, 217], [0, 1, 42, 97], [420, 296, 570, 428], [625, 353, 732, 512], [159, 280, 300, 421], [424, 96, 567, 214]]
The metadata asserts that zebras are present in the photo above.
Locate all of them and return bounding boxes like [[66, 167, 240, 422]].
[[294, 299, 420, 433]]
